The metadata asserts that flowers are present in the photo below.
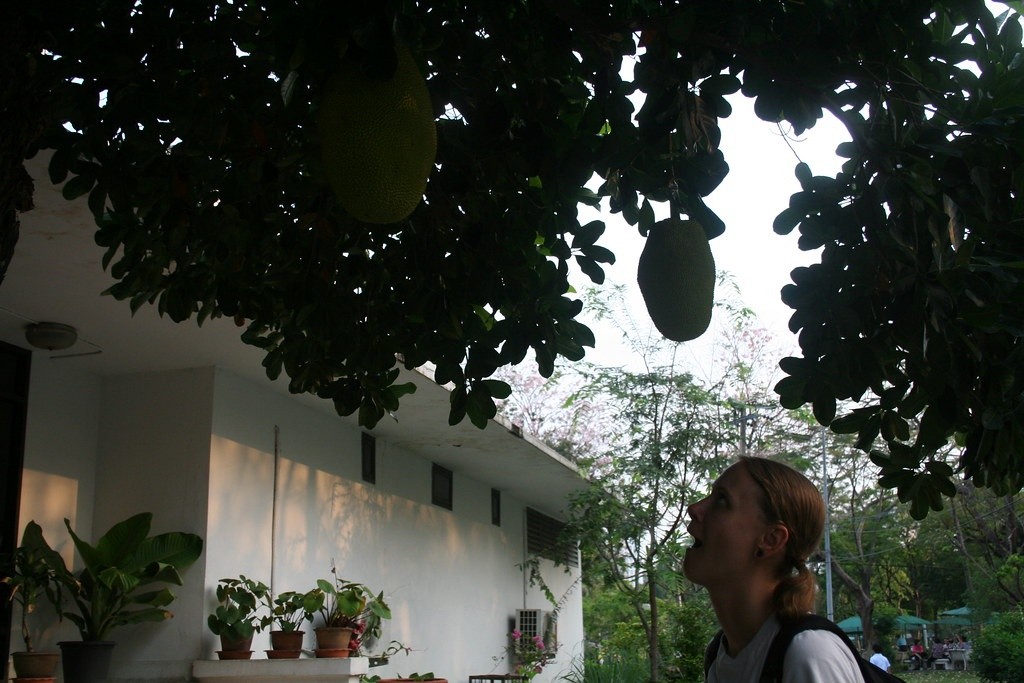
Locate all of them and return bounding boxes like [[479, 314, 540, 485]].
[[312, 557, 412, 658]]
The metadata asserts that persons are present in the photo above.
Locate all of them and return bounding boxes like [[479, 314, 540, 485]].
[[870, 643, 891, 674], [895, 635, 906, 649], [911, 639, 927, 671], [956, 636, 969, 651], [946, 639, 954, 649], [682, 457, 864, 683], [943, 637, 949, 659], [927, 638, 944, 670]]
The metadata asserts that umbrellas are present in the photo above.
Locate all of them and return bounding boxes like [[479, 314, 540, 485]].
[[893, 616, 933, 630], [836, 615, 878, 639], [933, 617, 992, 625], [938, 606, 971, 616]]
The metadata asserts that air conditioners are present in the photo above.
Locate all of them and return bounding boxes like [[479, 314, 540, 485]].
[[515, 607, 557, 654]]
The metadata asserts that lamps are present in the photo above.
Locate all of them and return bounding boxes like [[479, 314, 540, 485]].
[[26, 322, 78, 350]]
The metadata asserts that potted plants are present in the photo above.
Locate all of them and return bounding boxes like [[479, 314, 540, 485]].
[[208, 575, 274, 658], [260, 587, 325, 658], [49, 512, 204, 682], [1, 520, 68, 683], [359, 672, 447, 682]]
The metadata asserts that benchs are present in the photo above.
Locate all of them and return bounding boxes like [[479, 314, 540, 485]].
[[948, 648, 974, 670], [903, 658, 949, 669]]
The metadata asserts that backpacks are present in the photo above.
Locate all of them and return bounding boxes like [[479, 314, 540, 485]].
[[704, 613, 907, 683]]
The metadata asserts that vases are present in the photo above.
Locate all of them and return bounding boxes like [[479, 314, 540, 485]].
[[311, 627, 352, 659]]
